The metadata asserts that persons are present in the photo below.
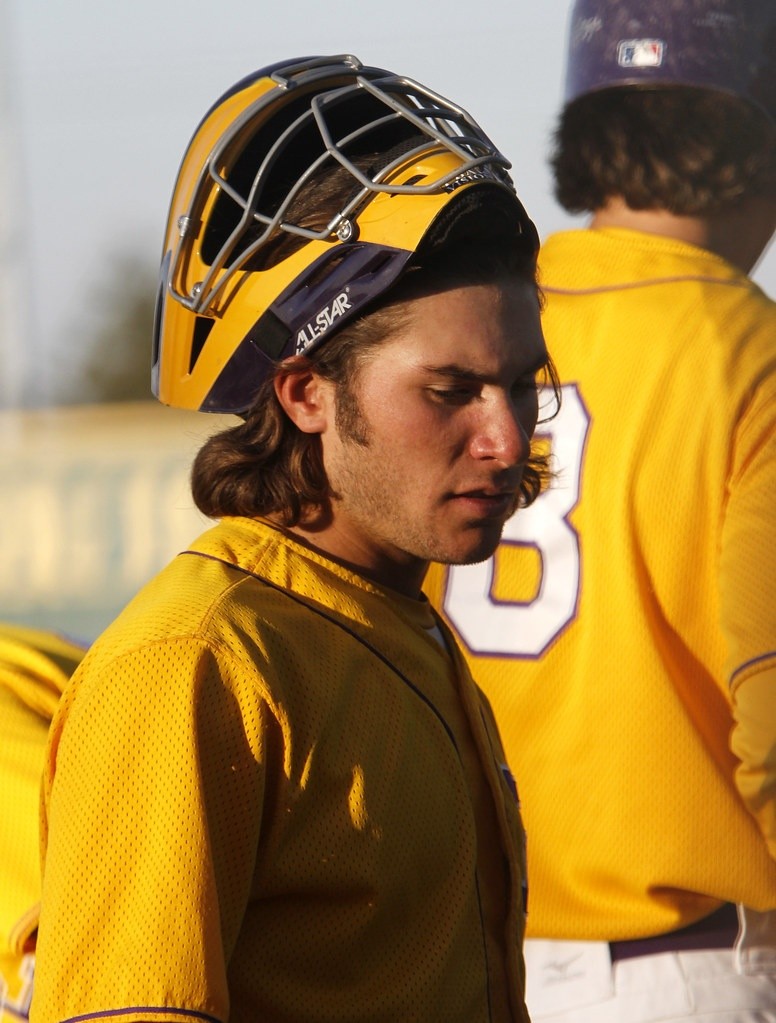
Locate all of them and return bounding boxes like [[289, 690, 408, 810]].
[[423, 0, 776, 1023], [38, 54, 560, 1023], [0, 625, 105, 1023]]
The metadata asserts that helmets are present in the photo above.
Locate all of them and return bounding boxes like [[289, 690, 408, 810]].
[[150, 53, 516, 416], [567, 0, 776, 108]]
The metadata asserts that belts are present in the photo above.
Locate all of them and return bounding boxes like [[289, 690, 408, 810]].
[[609, 899, 741, 961]]
